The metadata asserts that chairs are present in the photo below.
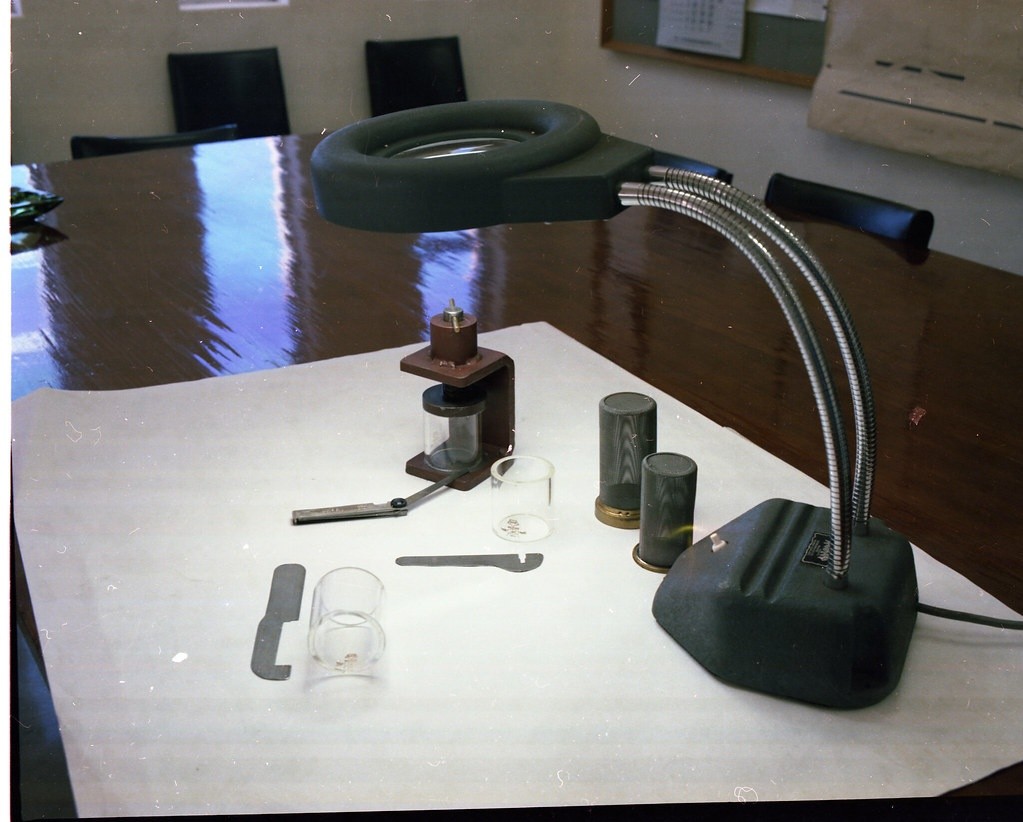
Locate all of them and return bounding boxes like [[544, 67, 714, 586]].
[[363, 33, 470, 116], [167, 47, 290, 142]]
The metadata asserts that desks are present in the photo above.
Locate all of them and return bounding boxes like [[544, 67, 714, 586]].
[[12, 128, 1022, 820]]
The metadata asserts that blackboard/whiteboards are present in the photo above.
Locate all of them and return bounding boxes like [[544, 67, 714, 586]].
[[600, 0, 831, 89]]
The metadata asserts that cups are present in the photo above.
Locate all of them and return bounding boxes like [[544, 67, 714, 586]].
[[490, 457, 555, 541], [306, 567, 386, 672], [422, 406, 482, 472]]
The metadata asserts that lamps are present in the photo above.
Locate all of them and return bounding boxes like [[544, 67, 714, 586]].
[[306, 95, 921, 713]]
